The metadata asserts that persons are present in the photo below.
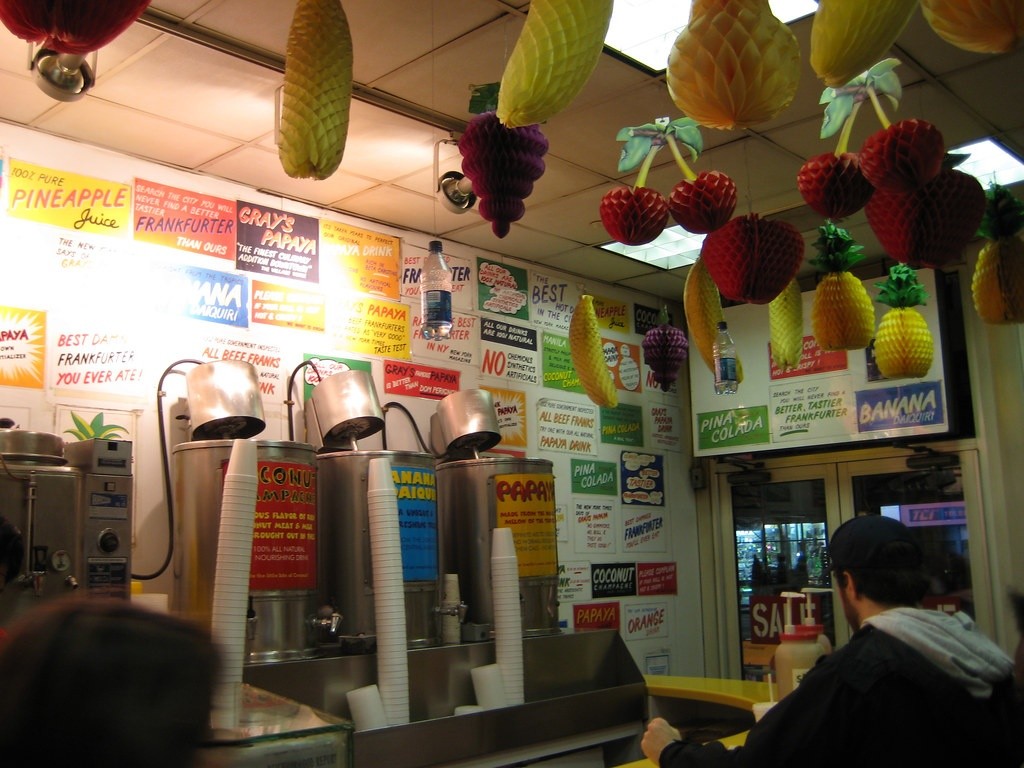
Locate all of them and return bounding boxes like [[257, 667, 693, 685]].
[[640, 515, 1024, 768], [0, 599, 225, 768]]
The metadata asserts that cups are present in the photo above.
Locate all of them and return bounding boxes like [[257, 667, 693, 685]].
[[210, 440, 258, 730], [346, 458, 410, 731], [444, 529, 524, 719]]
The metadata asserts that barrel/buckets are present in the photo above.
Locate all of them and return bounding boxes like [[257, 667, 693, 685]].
[[435, 457, 558, 637], [171, 441, 323, 665], [712, 321, 737, 395], [316, 450, 441, 651]]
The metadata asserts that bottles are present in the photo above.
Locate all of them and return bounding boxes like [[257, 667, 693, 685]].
[[419, 241, 453, 340]]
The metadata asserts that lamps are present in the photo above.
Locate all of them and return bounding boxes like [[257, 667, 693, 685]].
[[432, 138, 478, 214], [28, 37, 98, 102]]
